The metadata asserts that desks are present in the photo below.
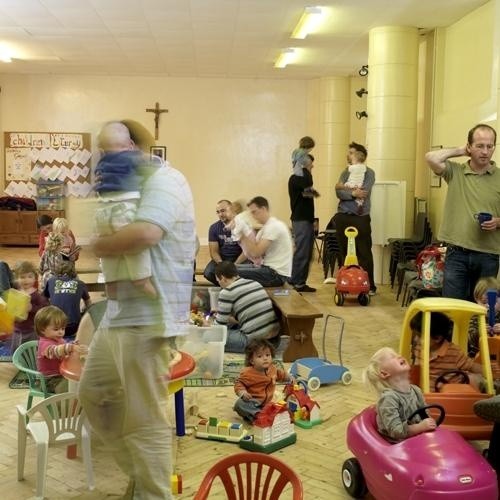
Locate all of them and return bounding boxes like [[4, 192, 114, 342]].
[[60, 340, 196, 437]]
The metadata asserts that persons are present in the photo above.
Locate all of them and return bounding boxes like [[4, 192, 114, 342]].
[[80, 120, 194, 499], [425, 124, 500, 302], [35, 305, 87, 393], [203, 199, 238, 288], [46, 218, 74, 255], [344, 151, 367, 215], [366, 347, 436, 441], [411, 310, 483, 391], [288, 155, 316, 292], [468, 278, 500, 356], [35, 213, 53, 256], [292, 137, 320, 196], [235, 196, 293, 290], [94, 122, 164, 300], [44, 261, 92, 335], [234, 338, 297, 424], [223, 203, 263, 267], [13, 261, 50, 341], [336, 142, 377, 296], [211, 262, 280, 350]]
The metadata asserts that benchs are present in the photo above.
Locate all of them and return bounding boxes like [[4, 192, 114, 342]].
[[75, 266, 103, 273], [264, 281, 324, 362]]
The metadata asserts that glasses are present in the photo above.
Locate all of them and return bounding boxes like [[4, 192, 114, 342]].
[[470, 144, 496, 150]]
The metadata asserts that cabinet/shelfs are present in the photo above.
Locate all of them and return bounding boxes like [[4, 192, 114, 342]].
[[1, 210, 66, 246]]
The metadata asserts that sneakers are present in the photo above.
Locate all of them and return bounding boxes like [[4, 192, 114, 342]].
[[369, 288, 375, 296], [303, 192, 313, 197]]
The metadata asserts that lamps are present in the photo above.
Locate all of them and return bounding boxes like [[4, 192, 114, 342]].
[[356, 111, 368, 120], [358, 65, 368, 76], [355, 87, 368, 98]]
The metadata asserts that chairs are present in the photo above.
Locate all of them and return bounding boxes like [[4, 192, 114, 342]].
[[11, 341, 77, 431], [15, 392, 97, 499], [319, 227, 342, 281], [387, 212, 436, 305], [193, 452, 304, 500]]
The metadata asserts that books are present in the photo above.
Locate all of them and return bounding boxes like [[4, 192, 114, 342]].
[[272, 288, 290, 297]]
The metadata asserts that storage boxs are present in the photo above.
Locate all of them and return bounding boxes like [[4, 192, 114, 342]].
[[35, 180, 62, 197], [175, 324, 228, 379], [35, 196, 66, 211]]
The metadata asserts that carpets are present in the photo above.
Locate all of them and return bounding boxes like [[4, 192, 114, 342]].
[[6, 335, 315, 390]]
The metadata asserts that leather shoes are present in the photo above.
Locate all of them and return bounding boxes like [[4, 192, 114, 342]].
[[295, 285, 316, 293]]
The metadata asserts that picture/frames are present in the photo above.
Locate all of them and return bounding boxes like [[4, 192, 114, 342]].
[[150, 145, 167, 168]]
